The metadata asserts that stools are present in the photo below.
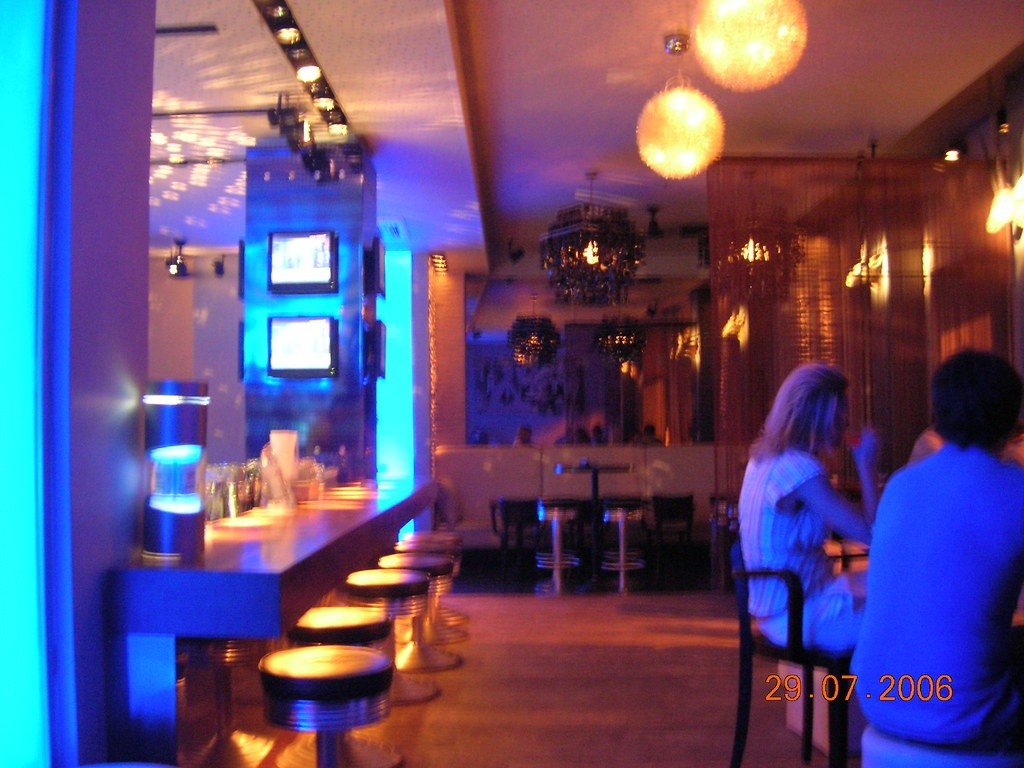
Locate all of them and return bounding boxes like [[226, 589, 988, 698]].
[[598, 492, 695, 598], [375, 529, 469, 673], [183, 640, 267, 767], [286, 607, 402, 767], [256, 644, 393, 768], [494, 495, 581, 595], [341, 567, 441, 704], [861, 723, 1024, 768], [729, 540, 854, 768]]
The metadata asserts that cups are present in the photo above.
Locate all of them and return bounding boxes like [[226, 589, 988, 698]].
[[844, 424, 888, 482]]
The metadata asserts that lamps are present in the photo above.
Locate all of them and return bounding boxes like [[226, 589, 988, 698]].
[[635, 34, 726, 179], [506, 294, 560, 370], [268, 91, 345, 184], [538, 172, 645, 305], [593, 302, 649, 363], [689, 0, 807, 91]]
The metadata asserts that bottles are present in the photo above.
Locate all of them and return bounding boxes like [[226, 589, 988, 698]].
[[203, 444, 352, 519]]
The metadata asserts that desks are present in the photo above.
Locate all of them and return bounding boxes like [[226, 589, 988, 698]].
[[555, 461, 630, 590]]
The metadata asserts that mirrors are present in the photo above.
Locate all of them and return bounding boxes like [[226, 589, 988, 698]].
[[463, 269, 715, 449]]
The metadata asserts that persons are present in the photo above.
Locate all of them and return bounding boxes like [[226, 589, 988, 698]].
[[552, 421, 666, 445], [905, 423, 942, 466], [512, 423, 536, 447], [473, 430, 490, 445], [850, 346, 1024, 768], [738, 362, 884, 654]]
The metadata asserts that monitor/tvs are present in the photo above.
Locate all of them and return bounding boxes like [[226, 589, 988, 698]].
[[266, 315, 337, 378], [373, 320, 387, 379], [267, 230, 338, 294], [374, 237, 386, 300]]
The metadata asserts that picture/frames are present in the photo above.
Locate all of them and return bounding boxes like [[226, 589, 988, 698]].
[[267, 315, 336, 378], [267, 229, 339, 292]]
